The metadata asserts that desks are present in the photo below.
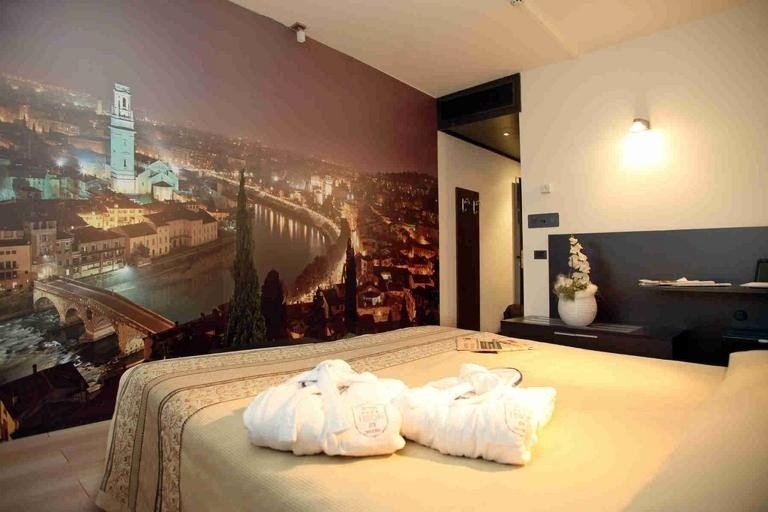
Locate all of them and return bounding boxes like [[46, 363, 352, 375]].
[[639, 286, 768, 360]]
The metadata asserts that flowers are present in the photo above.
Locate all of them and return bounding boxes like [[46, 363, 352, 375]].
[[555, 236, 592, 300]]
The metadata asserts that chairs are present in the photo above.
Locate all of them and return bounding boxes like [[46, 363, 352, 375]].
[[724, 258, 768, 364]]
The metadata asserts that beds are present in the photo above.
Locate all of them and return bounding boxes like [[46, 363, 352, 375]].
[[91, 326, 768, 512]]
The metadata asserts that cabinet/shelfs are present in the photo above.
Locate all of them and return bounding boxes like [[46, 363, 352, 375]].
[[499, 316, 652, 358]]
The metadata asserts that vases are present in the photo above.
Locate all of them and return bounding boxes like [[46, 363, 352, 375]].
[[558, 284, 598, 327]]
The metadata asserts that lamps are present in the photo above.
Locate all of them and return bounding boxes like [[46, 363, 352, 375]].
[[291, 22, 305, 43], [630, 119, 650, 131]]
